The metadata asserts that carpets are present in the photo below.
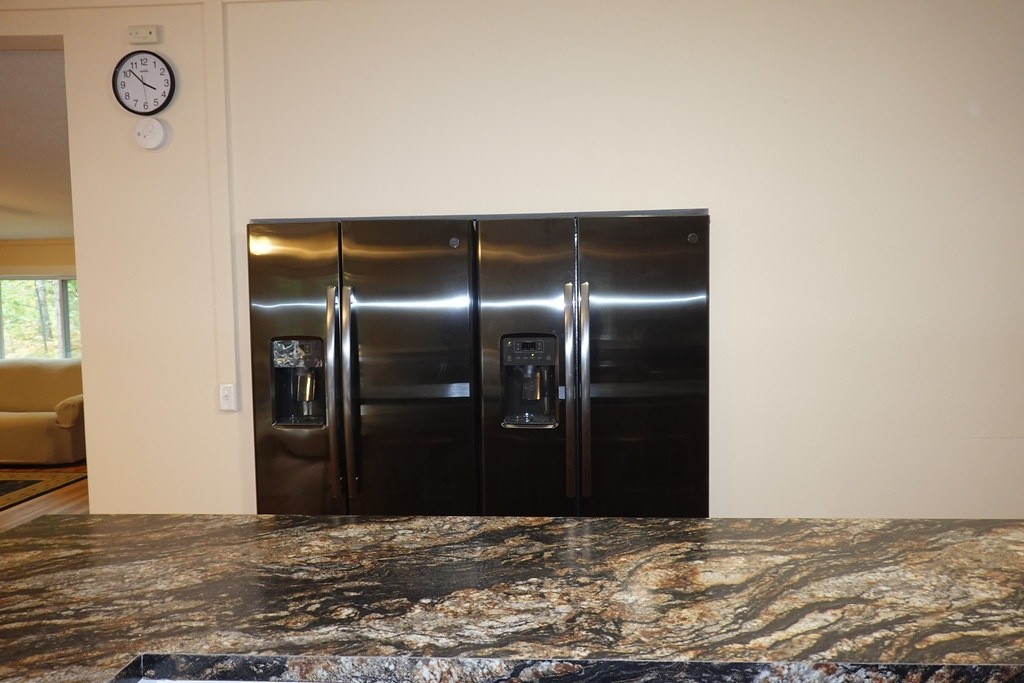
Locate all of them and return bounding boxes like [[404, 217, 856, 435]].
[[0, 472, 88, 512]]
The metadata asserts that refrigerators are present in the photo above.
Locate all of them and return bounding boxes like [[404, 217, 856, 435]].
[[246, 208, 709, 516]]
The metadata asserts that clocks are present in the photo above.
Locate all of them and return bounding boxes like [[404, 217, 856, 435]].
[[111, 49, 175, 117]]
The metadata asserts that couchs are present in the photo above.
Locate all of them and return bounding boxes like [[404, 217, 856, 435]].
[[0, 355, 84, 463]]
